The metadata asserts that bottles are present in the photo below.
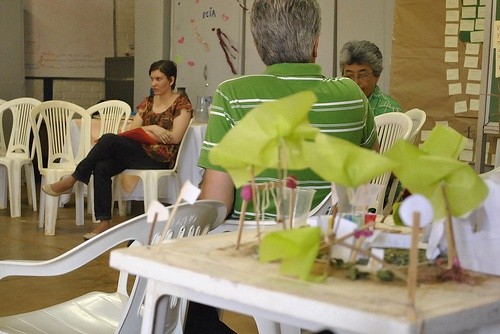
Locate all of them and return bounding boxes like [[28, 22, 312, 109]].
[[194, 96, 212, 123], [177, 88, 192, 118]]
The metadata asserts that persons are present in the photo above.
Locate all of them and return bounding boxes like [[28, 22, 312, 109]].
[[339, 40, 404, 209], [42, 60, 194, 241], [197, 0, 379, 219]]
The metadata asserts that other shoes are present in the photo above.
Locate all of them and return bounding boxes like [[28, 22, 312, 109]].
[[84, 232, 98, 240], [41, 184, 73, 196]]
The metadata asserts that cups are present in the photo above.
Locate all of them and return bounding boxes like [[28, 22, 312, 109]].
[[276, 187, 317, 229]]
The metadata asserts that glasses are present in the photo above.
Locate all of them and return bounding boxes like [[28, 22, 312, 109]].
[[342, 71, 375, 82]]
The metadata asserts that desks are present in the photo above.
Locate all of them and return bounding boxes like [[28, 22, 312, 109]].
[[109, 212, 500, 334], [59, 119, 208, 214]]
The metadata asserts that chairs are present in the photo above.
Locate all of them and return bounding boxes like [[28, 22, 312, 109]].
[[0, 199, 227, 334], [210, 108, 427, 334], [0, 98, 195, 237]]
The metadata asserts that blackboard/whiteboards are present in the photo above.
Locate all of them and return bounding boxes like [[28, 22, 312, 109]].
[[477, 0, 500, 136]]
